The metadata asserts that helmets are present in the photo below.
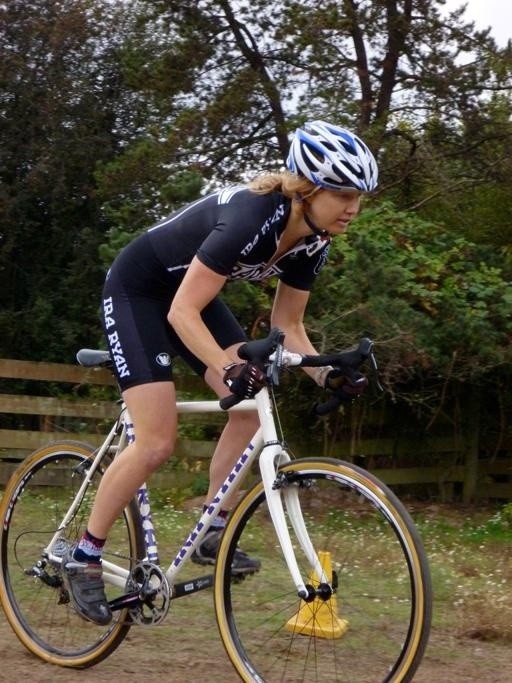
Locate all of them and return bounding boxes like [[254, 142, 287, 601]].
[[285, 119, 378, 193]]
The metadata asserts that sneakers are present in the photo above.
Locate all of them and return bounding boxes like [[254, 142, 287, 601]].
[[191, 529, 261, 574], [61, 550, 113, 625]]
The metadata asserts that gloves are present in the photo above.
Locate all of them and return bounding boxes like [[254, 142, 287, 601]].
[[325, 370, 369, 400], [223, 363, 267, 399]]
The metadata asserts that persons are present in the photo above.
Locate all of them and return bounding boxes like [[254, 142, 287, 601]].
[[62, 116, 380, 625]]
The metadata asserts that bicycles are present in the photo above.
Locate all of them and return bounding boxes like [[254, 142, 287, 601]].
[[0, 329, 431, 683]]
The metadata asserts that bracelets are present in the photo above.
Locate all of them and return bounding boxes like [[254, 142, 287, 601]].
[[314, 363, 330, 389]]
